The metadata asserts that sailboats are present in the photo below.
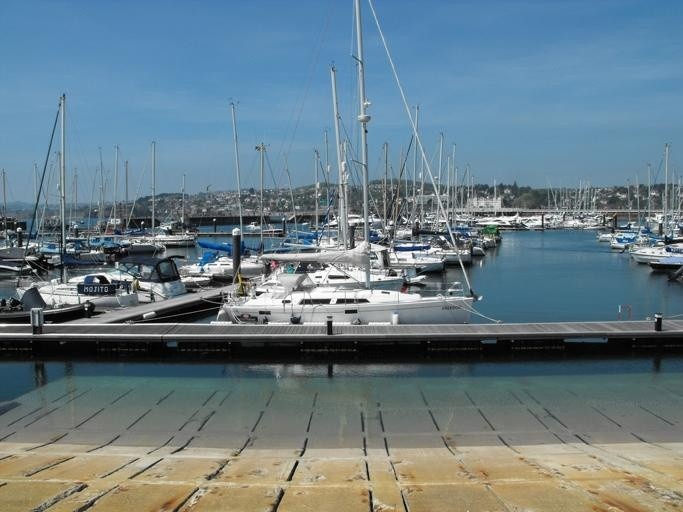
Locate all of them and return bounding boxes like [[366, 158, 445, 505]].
[[0, 0, 616, 326], [611, 145, 683, 277]]
